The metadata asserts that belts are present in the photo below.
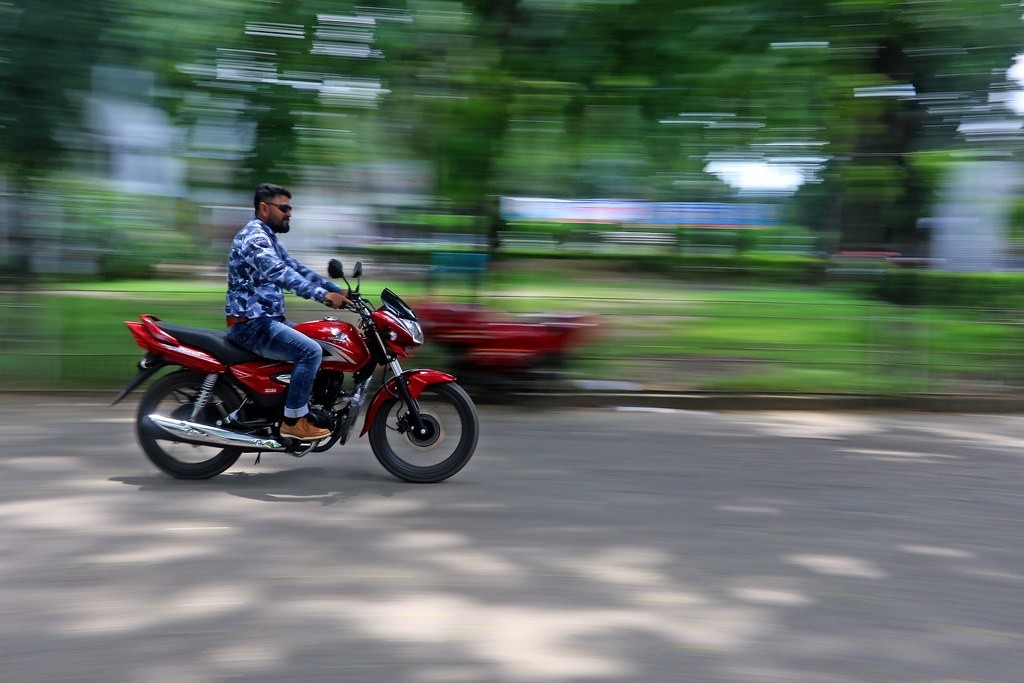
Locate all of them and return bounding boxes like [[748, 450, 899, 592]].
[[227, 316, 247, 326]]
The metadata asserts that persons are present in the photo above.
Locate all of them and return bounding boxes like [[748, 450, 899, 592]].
[[224, 183, 356, 441]]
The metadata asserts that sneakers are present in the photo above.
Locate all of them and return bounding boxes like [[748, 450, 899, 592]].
[[281, 417, 331, 441]]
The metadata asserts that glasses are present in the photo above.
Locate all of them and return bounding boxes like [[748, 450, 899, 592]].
[[264, 200, 293, 213]]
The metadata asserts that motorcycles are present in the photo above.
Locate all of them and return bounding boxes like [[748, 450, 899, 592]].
[[388, 294, 594, 402], [112, 259, 481, 484]]
